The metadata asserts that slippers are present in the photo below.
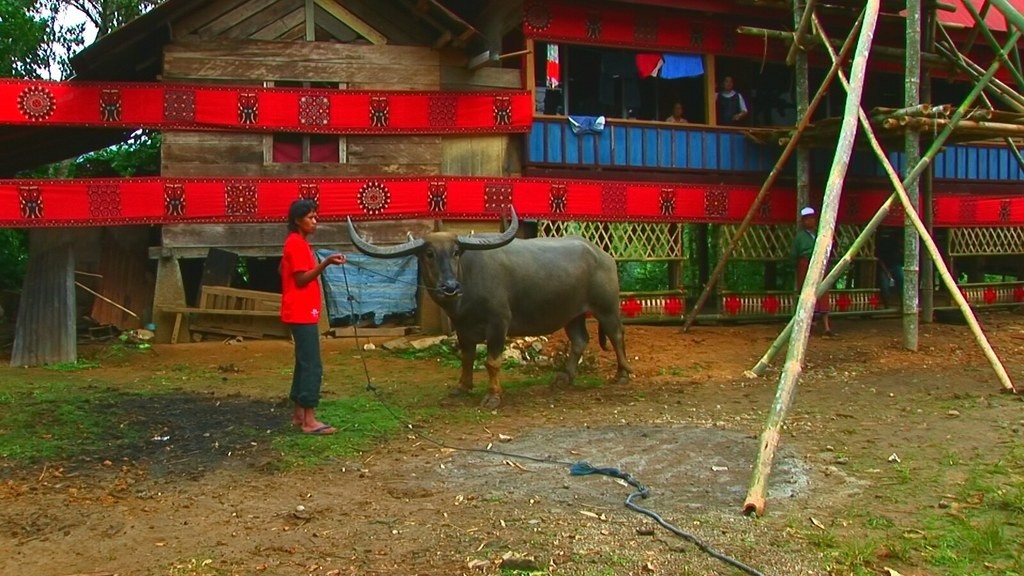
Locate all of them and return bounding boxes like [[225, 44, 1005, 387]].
[[292, 421, 302, 427], [300, 425, 337, 435]]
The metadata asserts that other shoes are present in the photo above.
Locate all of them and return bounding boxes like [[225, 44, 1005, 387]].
[[825, 329, 831, 335]]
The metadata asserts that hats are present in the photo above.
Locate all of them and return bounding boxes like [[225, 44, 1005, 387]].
[[801, 207, 814, 216]]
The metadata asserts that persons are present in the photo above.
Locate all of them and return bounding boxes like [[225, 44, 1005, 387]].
[[666, 104, 688, 123], [790, 207, 836, 340], [715, 77, 748, 124], [280, 197, 346, 435], [874, 248, 892, 309]]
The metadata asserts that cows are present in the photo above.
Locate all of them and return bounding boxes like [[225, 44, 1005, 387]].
[[349, 205, 634, 411]]
